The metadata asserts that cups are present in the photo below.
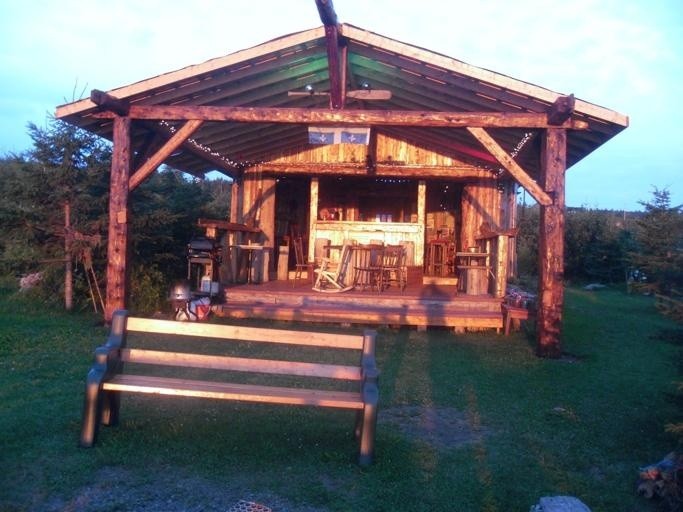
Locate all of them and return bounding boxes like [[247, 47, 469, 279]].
[[323, 212, 328, 222]]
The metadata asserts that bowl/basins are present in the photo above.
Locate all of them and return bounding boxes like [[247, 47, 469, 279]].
[[467, 247, 479, 253]]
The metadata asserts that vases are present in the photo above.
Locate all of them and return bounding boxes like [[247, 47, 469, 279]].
[[513, 300, 520, 308]]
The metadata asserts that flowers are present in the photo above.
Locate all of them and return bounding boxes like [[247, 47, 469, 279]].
[[511, 289, 528, 301]]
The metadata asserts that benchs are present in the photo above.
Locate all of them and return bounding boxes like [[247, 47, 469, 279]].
[[78, 309, 380, 468]]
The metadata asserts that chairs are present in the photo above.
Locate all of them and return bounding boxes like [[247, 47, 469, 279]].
[[293, 236, 315, 288], [187, 236, 216, 280], [354, 242, 405, 294], [312, 243, 354, 295]]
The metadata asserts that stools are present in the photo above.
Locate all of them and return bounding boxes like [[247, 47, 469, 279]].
[[429, 238, 447, 278], [502, 304, 528, 337]]
